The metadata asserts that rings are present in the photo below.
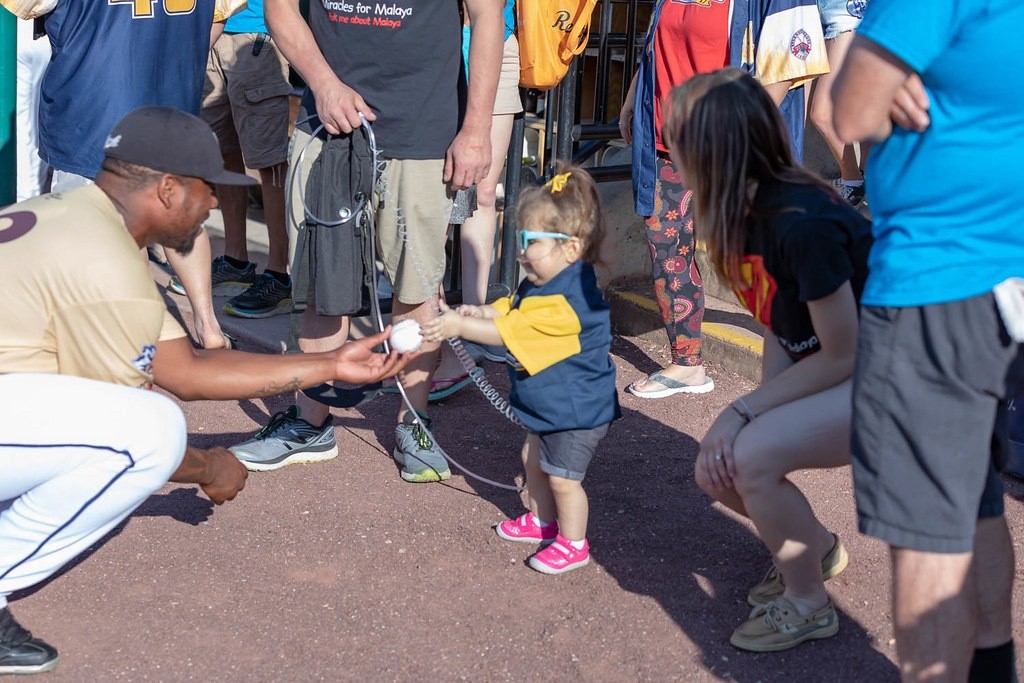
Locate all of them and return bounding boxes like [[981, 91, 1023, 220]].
[[714, 455, 722, 460]]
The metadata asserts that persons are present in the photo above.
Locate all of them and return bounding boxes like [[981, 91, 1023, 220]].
[[830, 0, 1023, 683], [38, 0, 238, 350], [16, 17, 52, 203], [169, 0, 294, 320], [226, 0, 510, 483], [663, 67, 874, 651], [420, 163, 622, 576], [0, 105, 418, 675], [381, 0, 524, 401], [809, 0, 872, 205], [618, 0, 830, 399]]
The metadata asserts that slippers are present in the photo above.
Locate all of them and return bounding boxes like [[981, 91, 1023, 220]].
[[428, 366, 485, 401], [629, 366, 715, 399], [196, 333, 238, 351], [379, 374, 402, 394]]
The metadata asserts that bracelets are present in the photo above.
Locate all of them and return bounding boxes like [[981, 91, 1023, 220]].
[[731, 396, 756, 422]]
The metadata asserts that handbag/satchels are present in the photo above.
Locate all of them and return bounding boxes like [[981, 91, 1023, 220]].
[[516, 0, 598, 89]]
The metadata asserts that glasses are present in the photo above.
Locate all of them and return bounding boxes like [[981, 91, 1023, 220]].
[[515, 230, 570, 251]]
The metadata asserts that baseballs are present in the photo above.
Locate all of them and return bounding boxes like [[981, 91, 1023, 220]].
[[389, 318, 423, 355]]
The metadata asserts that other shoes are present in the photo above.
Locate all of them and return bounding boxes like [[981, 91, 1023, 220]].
[[351, 291, 393, 316], [462, 339, 507, 363], [295, 300, 306, 312]]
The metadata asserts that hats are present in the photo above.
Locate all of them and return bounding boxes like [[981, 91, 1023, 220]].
[[104, 104, 259, 186]]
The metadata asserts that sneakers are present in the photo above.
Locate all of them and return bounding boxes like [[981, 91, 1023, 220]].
[[222, 273, 295, 319], [0, 606, 59, 675], [729, 594, 838, 652], [747, 531, 849, 606], [169, 255, 262, 297], [826, 169, 866, 210], [393, 409, 451, 483], [496, 512, 559, 545], [528, 533, 590, 575], [225, 405, 338, 472]]
